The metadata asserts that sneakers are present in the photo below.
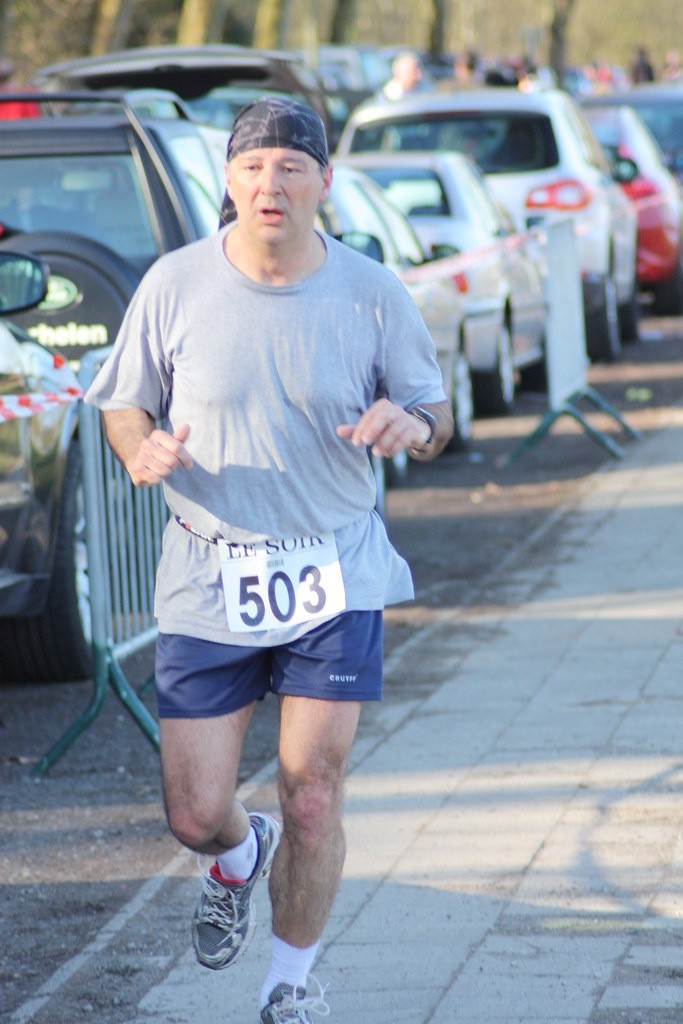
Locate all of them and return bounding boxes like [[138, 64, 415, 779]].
[[190, 812, 280, 970], [259, 972, 330, 1024]]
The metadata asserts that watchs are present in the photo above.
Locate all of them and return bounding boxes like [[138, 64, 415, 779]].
[[406, 407, 437, 455]]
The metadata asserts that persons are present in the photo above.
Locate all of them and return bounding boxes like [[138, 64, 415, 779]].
[[85, 96, 453, 1024], [384, 47, 683, 99]]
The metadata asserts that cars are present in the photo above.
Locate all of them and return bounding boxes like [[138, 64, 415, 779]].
[[1, 249, 111, 680], [30, 35, 683, 171], [321, 149, 559, 453], [585, 104, 682, 317]]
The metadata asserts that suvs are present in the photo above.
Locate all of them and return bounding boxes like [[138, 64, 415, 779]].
[[340, 74, 651, 361], [0, 86, 229, 302]]
